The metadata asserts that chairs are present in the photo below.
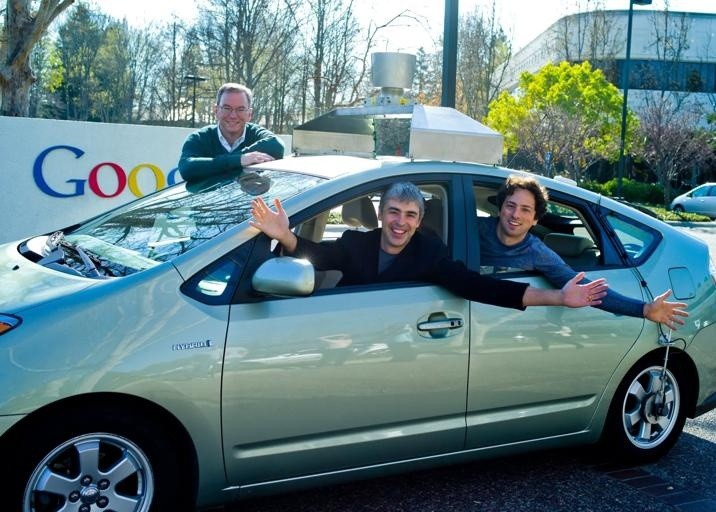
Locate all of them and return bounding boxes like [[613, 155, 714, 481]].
[[530, 223, 599, 268], [340, 195, 442, 240]]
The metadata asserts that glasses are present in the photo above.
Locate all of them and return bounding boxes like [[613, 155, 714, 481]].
[[217, 105, 248, 113]]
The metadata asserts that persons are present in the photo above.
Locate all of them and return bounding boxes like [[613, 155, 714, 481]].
[[177, 82, 286, 183], [247, 182, 611, 312], [473, 174, 690, 331]]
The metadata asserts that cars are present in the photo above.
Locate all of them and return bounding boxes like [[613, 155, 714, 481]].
[[0, 156, 716, 512]]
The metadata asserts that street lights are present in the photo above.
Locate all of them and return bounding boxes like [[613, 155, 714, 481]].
[[185, 74, 209, 127]]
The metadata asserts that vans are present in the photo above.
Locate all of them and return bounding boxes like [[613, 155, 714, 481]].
[[665, 178, 716, 218]]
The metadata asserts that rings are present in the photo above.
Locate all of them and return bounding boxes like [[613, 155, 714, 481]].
[[587, 294, 593, 303]]
